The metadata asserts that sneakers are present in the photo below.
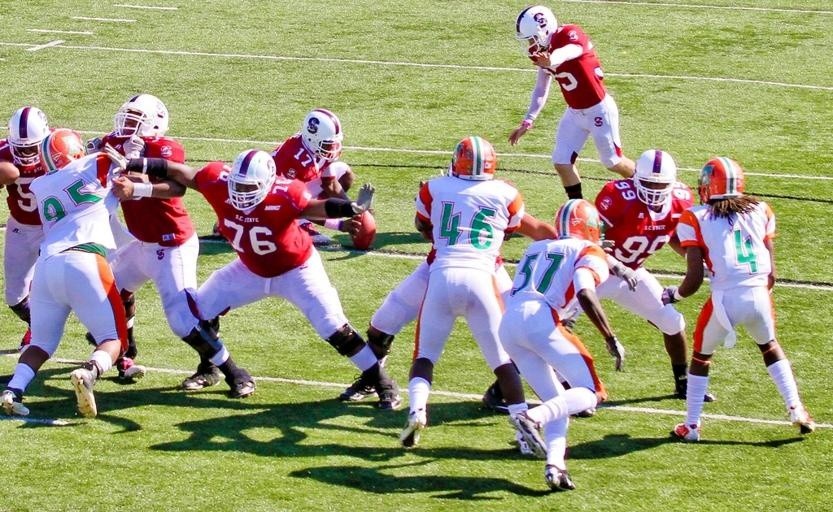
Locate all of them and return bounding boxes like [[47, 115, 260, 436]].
[[67, 363, 101, 423], [120, 361, 146, 384], [339, 377, 373, 403], [484, 387, 512, 414], [0, 389, 31, 417], [180, 367, 224, 391], [376, 384, 402, 410], [399, 414, 426, 450], [226, 372, 256, 399], [510, 410, 548, 459], [787, 407, 816, 435], [672, 420, 700, 443], [515, 431, 537, 455], [676, 380, 717, 403], [543, 464, 577, 490], [19, 327, 33, 354]]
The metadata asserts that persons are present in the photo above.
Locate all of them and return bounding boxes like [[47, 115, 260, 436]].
[[481, 147, 715, 403], [660, 156, 816, 444], [341, 181, 615, 401], [1, 129, 146, 419], [213, 108, 376, 250], [510, 4, 637, 200], [103, 144, 402, 413], [498, 199, 626, 489], [96, 95, 255, 399], [402, 135, 548, 459], [0, 106, 146, 381]]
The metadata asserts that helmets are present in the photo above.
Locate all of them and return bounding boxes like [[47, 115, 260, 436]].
[[39, 129, 86, 174], [226, 148, 277, 211], [7, 105, 52, 168], [114, 92, 170, 139], [450, 134, 498, 182], [515, 1, 558, 56], [553, 197, 605, 245], [633, 148, 679, 206], [699, 157, 745, 200], [300, 108, 345, 162]]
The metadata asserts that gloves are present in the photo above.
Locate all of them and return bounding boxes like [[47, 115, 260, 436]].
[[606, 335, 624, 371], [661, 286, 682, 306], [123, 136, 146, 160], [105, 142, 128, 175], [351, 184, 376, 214], [612, 261, 638, 292]]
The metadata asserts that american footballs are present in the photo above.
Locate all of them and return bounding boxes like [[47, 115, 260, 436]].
[[0, 161, 20, 184], [351, 210, 376, 250]]
